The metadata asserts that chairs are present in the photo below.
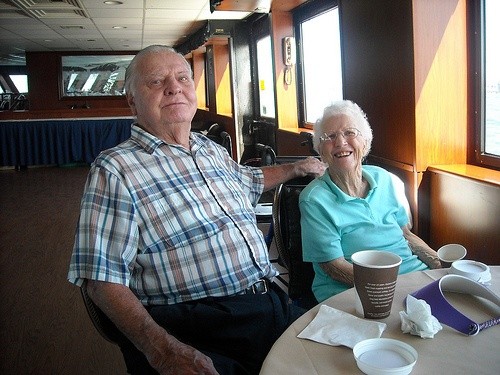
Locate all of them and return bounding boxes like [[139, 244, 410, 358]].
[[272, 175, 319, 309], [219, 131, 233, 160], [240, 144, 278, 167]]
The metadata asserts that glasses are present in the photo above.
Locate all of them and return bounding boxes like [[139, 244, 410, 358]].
[[318, 128, 361, 145]]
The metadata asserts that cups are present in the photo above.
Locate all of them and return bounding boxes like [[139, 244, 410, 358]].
[[351, 250, 402, 319], [437, 244, 468, 268]]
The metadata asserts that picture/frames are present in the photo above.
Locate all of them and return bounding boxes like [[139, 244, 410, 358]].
[[56, 50, 140, 101]]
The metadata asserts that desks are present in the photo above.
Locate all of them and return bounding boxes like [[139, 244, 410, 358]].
[[0, 115, 137, 171]]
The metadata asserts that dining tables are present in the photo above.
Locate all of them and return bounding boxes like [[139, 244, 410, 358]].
[[258, 265, 500, 375]]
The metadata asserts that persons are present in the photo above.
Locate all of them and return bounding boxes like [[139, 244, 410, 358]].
[[300, 99, 441, 303], [68, 45, 327, 375]]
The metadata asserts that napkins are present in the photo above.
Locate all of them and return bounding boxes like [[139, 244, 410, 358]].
[[298, 304, 388, 349]]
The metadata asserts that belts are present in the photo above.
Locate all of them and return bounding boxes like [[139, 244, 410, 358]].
[[180, 276, 271, 305]]
